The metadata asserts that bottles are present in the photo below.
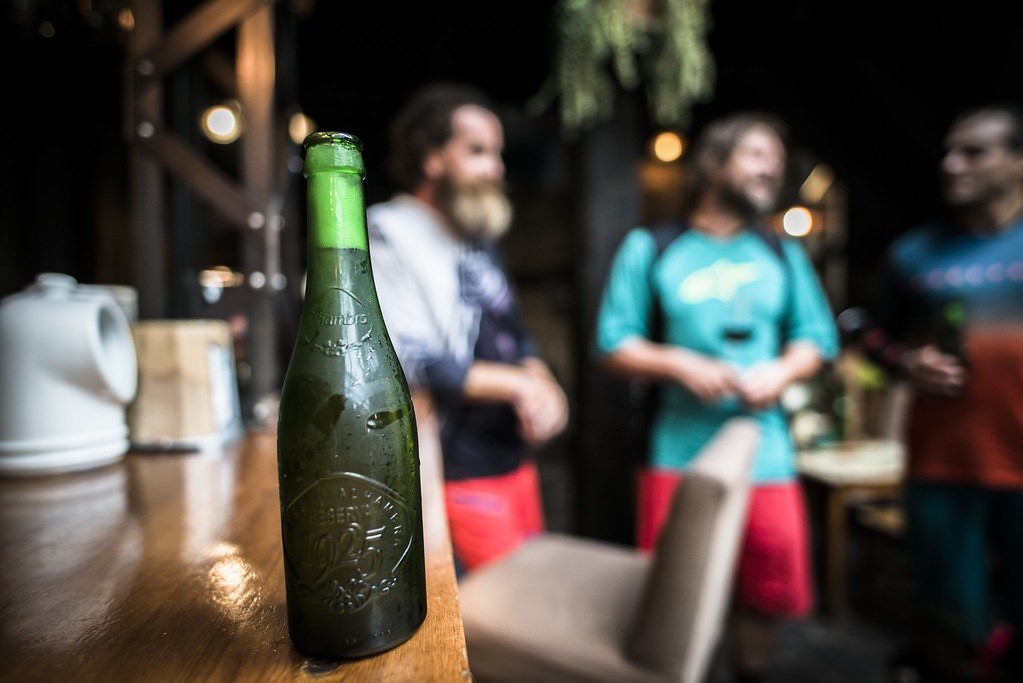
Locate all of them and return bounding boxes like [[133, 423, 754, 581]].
[[276, 132, 429, 669], [936, 292, 968, 357]]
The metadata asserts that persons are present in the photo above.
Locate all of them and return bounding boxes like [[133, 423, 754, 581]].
[[851, 105, 1023, 683], [300, 87, 570, 581], [591, 115, 842, 683]]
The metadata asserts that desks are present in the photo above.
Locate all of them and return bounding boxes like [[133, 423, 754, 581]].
[[797, 441, 914, 618], [1, 391, 470, 683]]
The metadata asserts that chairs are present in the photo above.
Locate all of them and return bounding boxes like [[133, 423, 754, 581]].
[[456, 415, 763, 683]]
